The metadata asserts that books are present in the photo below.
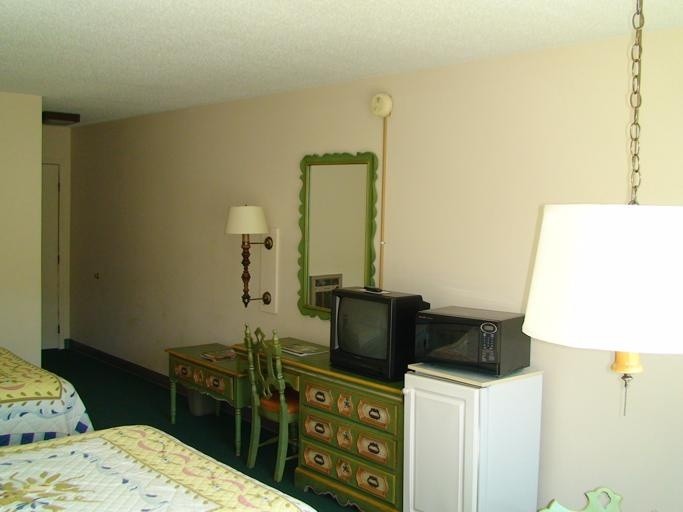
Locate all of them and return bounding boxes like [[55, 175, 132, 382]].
[[199, 348, 236, 363], [281, 344, 327, 357]]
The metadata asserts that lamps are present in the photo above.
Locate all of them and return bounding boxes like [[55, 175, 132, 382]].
[[521, 2, 682, 413], [223, 201, 276, 312]]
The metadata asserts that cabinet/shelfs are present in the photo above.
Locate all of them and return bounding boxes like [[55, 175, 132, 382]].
[[283, 370, 396, 507], [401, 362, 544, 512]]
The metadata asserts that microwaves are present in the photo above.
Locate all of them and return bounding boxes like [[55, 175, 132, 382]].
[[412, 303, 530, 377]]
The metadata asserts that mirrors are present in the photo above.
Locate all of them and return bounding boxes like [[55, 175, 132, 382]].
[[296, 151, 379, 322]]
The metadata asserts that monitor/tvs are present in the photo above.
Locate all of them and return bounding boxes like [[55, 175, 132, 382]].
[[330, 287, 430, 383]]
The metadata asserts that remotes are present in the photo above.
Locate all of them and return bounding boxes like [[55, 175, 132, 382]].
[[365, 286, 382, 292]]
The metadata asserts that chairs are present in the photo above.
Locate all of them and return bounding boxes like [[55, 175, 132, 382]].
[[241, 321, 303, 484]]
